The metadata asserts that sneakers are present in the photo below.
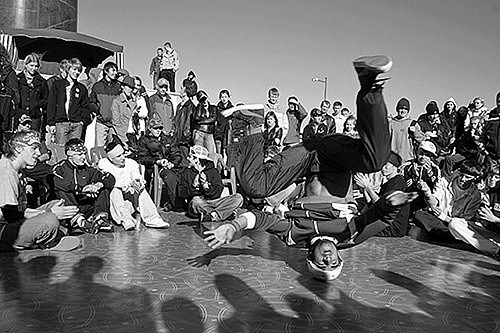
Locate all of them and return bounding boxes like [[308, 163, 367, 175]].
[[352, 55, 392, 84], [220, 104, 263, 126]]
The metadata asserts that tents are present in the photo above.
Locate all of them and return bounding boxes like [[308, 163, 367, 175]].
[[5, 29, 124, 71]]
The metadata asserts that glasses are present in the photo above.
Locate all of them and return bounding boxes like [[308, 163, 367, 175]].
[[151, 126, 163, 130], [22, 121, 32, 126]]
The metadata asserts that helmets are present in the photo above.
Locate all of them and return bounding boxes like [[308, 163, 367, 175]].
[[303, 254, 343, 281]]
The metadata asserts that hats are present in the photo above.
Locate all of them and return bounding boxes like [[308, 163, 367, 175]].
[[396, 98, 410, 112], [118, 76, 137, 90], [157, 78, 169, 87], [419, 141, 438, 157], [148, 117, 164, 127], [19, 115, 31, 123], [189, 145, 212, 161]]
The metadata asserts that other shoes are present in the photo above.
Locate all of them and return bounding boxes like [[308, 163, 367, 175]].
[[95, 220, 115, 231], [46, 236, 79, 252], [210, 213, 219, 221], [121, 220, 135, 230], [144, 218, 170, 228]]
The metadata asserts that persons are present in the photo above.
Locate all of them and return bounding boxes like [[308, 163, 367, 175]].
[[89, 62, 122, 147], [150, 48, 163, 90], [0, 33, 500, 261], [47, 58, 89, 164], [159, 41, 180, 93], [203, 54, 420, 280]]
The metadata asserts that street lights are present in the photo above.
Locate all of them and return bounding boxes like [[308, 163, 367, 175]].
[[311, 76, 327, 99]]
